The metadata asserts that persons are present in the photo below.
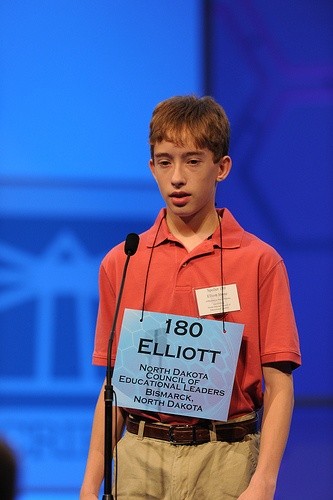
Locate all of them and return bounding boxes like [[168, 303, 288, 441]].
[[78, 94, 305, 500]]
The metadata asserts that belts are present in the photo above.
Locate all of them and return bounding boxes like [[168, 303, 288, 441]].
[[126, 414, 263, 447]]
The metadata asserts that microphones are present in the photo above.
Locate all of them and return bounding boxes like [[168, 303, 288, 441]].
[[123, 232, 140, 257]]
